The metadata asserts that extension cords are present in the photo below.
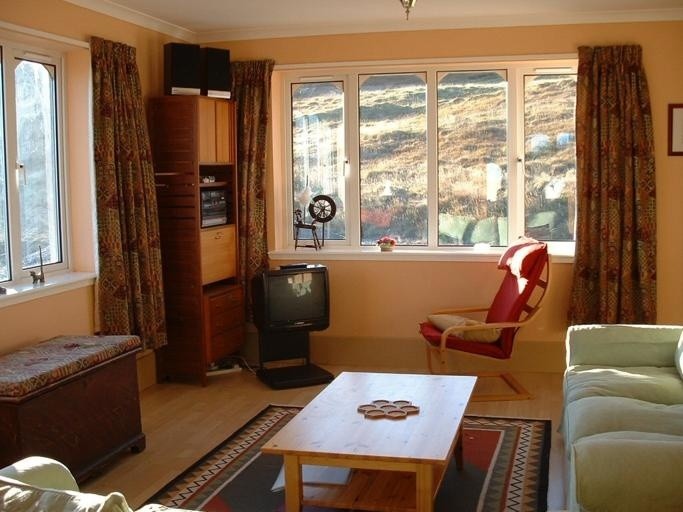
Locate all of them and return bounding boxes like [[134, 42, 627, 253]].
[[205, 367, 242, 376]]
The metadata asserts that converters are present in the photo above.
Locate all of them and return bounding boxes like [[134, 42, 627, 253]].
[[217, 356, 232, 369]]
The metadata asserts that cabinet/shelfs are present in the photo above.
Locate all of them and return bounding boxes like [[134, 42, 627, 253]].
[[204, 285, 247, 366], [147, 95, 244, 387]]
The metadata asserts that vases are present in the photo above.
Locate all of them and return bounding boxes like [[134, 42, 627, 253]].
[[379, 243, 394, 251]]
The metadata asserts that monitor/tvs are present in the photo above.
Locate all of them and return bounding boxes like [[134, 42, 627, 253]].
[[252, 264, 329, 331]]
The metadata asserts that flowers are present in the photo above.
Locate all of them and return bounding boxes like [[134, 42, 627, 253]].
[[373, 236, 396, 246]]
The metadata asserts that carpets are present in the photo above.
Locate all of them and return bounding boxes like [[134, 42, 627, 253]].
[[134, 402, 551, 512]]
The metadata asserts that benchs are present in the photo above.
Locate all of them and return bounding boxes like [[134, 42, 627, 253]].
[[0, 333, 145, 484]]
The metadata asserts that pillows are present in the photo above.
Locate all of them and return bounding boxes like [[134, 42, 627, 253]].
[[493, 238, 546, 279], [426, 313, 502, 344]]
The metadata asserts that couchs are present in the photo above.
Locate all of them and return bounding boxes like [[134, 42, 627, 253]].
[[557, 324, 682, 511], [0, 456, 204, 512]]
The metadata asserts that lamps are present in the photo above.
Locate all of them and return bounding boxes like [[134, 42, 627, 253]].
[[398, 0, 416, 22]]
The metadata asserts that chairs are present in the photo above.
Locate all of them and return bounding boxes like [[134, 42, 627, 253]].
[[415, 234, 551, 404]]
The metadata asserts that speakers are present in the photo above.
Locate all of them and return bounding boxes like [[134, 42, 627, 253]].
[[201, 47, 231, 99], [164, 42, 201, 95]]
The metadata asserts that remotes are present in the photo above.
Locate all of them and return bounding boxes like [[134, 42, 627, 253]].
[[280, 263, 308, 269]]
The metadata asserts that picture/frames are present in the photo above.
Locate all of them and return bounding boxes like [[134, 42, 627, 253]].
[[667, 103, 682, 156]]
